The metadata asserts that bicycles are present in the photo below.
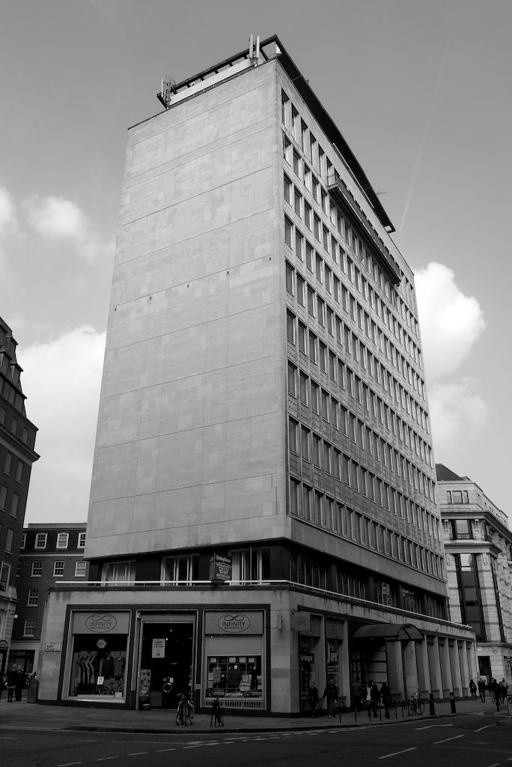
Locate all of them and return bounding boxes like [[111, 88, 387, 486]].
[[175, 690, 226, 728], [407, 687, 425, 716]]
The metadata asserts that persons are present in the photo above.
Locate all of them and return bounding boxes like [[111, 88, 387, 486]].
[[469, 676, 510, 712], [352, 675, 392, 719], [6, 663, 18, 702], [14, 664, 24, 701], [305, 680, 319, 718], [323, 677, 339, 719]]
